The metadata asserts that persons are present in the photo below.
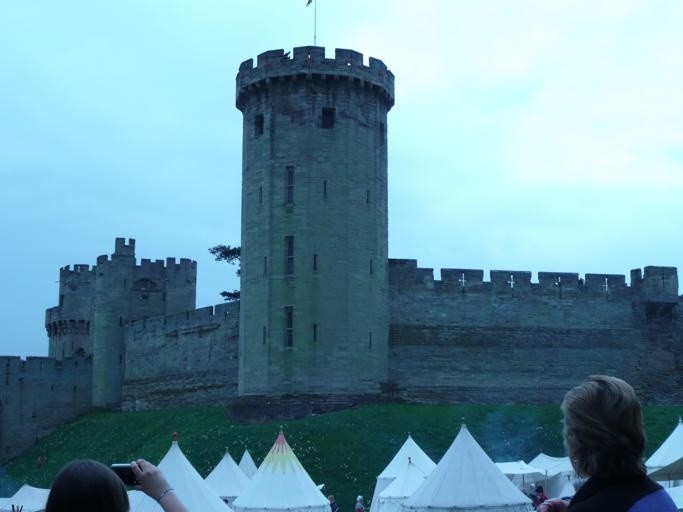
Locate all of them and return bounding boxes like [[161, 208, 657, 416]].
[[534, 373, 680, 512], [43, 456, 190, 511], [328, 495, 339, 512], [355, 495, 365, 512]]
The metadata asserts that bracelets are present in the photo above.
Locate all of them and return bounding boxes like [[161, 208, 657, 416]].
[[157, 489, 173, 503]]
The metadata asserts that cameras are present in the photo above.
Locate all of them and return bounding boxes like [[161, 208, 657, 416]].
[[112, 464, 143, 486]]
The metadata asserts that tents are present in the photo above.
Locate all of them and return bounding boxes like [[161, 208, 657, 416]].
[[200, 447, 253, 511], [124, 431, 233, 512], [229, 425, 332, 512], [0, 480, 53, 511], [237, 447, 260, 482]]
